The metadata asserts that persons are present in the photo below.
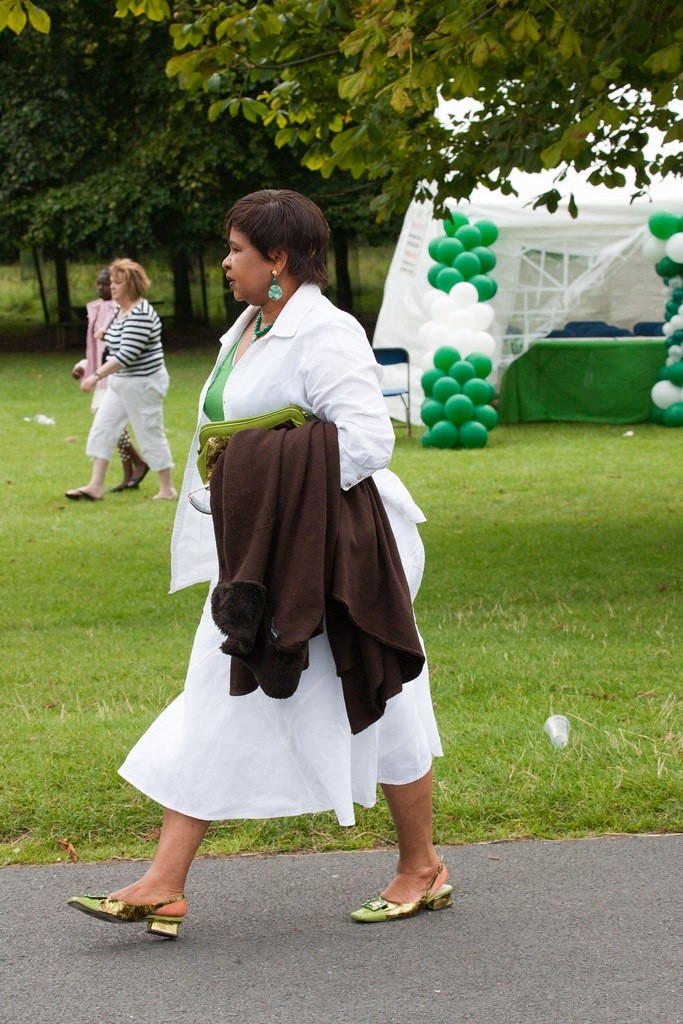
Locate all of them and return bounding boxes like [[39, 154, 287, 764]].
[[65, 189, 453, 937]]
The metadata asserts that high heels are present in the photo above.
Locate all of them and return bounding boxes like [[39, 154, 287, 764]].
[[349, 858, 454, 922], [68, 895, 186, 938]]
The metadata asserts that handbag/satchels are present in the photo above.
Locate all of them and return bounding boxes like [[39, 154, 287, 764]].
[[196, 405, 310, 491]]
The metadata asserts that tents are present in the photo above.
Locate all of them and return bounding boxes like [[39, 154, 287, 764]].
[[371, 79, 683, 427]]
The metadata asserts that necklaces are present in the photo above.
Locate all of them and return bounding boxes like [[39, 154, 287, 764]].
[[246, 310, 277, 347]]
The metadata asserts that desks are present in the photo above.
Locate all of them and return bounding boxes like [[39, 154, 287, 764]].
[[498, 336, 666, 422], [55, 301, 165, 320]]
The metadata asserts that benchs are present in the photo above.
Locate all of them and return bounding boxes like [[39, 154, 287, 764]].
[[54, 321, 87, 351]]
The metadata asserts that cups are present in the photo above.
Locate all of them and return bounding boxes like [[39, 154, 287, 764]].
[[544, 715, 571, 746]]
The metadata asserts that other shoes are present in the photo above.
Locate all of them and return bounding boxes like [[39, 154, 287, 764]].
[[127, 465, 149, 487], [110, 483, 137, 493]]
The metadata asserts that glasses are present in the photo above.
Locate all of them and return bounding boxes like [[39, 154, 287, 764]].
[[188, 485, 210, 514]]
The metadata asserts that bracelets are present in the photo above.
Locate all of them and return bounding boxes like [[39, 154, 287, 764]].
[[91, 371, 103, 380]]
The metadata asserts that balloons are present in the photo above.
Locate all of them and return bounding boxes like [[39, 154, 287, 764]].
[[421, 212, 499, 449], [648, 212, 683, 428]]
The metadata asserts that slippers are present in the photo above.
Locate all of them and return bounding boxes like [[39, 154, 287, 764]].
[[64, 490, 94, 500]]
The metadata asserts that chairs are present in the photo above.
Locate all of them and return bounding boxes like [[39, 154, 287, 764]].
[[547, 320, 666, 337], [371, 347, 412, 437]]
